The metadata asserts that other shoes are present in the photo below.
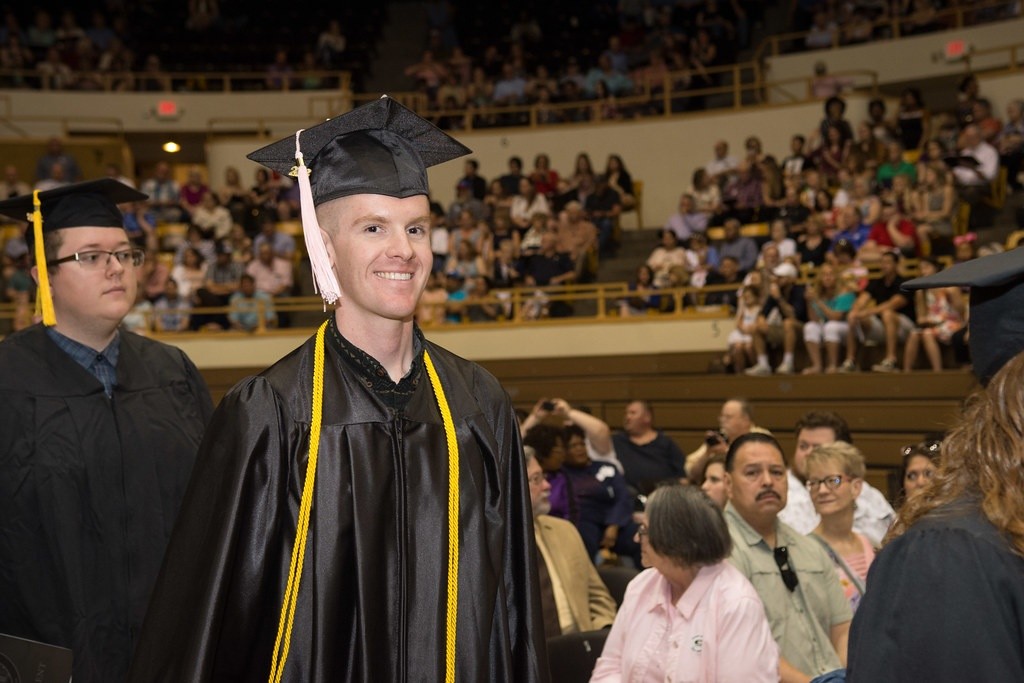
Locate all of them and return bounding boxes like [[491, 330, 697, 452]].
[[747, 362, 902, 377]]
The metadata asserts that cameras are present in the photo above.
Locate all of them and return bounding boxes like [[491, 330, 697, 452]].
[[707, 434, 729, 447]]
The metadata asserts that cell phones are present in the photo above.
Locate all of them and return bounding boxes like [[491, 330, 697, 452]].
[[542, 402, 554, 410]]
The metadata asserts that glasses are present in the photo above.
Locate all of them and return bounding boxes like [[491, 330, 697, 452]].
[[638, 525, 649, 535], [901, 441, 942, 457], [806, 474, 850, 491], [48, 247, 147, 268]]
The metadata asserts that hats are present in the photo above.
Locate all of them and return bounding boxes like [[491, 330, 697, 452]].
[[893, 244, 1024, 385], [246, 93, 473, 314], [0, 178, 151, 330]]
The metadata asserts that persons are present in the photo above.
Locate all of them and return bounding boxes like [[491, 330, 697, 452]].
[[804, 441, 876, 614], [0, 0, 1024, 131], [776, 406, 897, 550], [0, 179, 217, 683], [414, 152, 638, 326], [523, 445, 617, 639], [900, 440, 942, 496], [589, 485, 781, 683], [0, 137, 303, 332], [513, 397, 772, 571], [842, 352, 1024, 683], [624, 71, 1024, 378], [722, 432, 854, 683], [124, 95, 553, 683]]
